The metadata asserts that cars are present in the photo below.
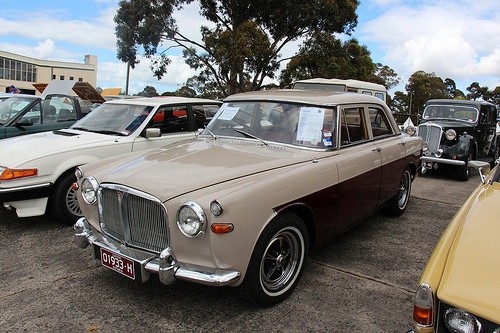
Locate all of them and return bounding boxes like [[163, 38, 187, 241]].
[[411, 159, 499, 333], [0, 93, 94, 139], [416, 98, 500, 180], [72, 90, 424, 309], [0, 96, 273, 226]]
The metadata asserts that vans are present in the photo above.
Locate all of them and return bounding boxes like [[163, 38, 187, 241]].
[[290, 78, 387, 104]]
